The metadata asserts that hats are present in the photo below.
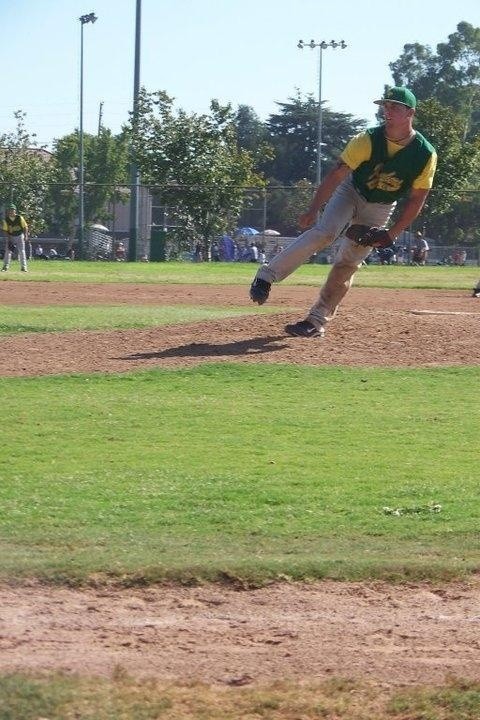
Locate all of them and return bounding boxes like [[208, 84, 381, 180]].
[[373, 86, 417, 110]]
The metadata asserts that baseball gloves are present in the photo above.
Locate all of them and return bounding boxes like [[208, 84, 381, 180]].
[[7, 242, 16, 254], [346, 225, 393, 248]]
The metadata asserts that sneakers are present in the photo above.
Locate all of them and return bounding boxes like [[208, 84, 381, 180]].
[[249, 277, 271, 305], [285, 320, 325, 339]]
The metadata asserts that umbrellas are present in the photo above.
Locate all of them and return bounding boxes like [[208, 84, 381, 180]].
[[236, 228, 258, 235], [259, 229, 280, 236]]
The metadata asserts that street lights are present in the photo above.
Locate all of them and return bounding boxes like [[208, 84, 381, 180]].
[[296, 40, 348, 265], [78, 12, 98, 262]]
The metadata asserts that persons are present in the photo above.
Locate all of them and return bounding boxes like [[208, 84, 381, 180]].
[[36, 244, 47, 259], [409, 232, 429, 265], [249, 88, 438, 338], [2, 204, 28, 273], [116, 241, 125, 261], [196, 237, 266, 265], [49, 246, 56, 257]]
